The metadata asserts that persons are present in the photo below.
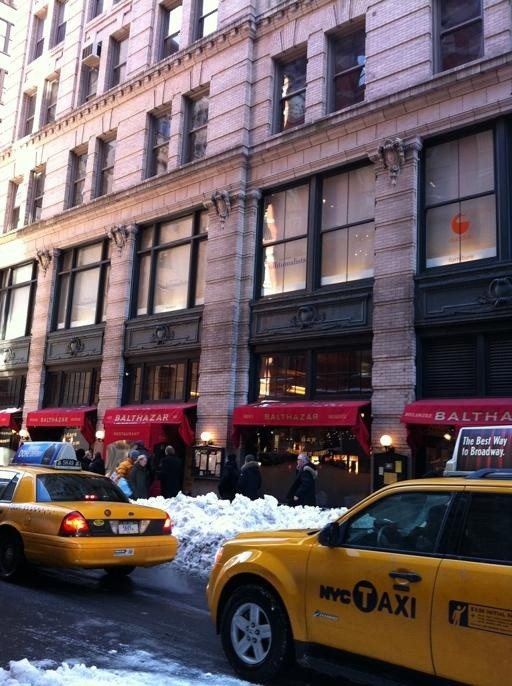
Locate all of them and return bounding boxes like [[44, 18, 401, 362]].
[[73, 439, 184, 500], [219, 452, 240, 504], [372, 504, 444, 556], [239, 454, 264, 501], [286, 452, 320, 506]]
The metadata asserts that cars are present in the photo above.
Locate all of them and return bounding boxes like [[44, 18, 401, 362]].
[[205, 425, 512, 685], [0, 441, 178, 583]]
